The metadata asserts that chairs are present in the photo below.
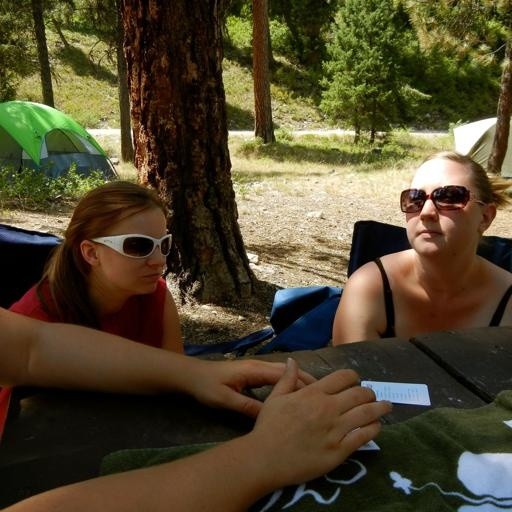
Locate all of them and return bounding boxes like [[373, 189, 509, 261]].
[[0, 224, 274, 358], [257, 220, 512, 357]]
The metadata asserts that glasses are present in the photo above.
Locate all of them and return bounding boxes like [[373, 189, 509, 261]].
[[85, 226, 175, 260], [398, 186, 487, 216]]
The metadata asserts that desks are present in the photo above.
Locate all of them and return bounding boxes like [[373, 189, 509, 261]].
[[0, 324, 512, 512]]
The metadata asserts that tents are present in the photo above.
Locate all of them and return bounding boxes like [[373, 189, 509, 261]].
[[0, 99, 123, 182], [452, 114, 512, 180]]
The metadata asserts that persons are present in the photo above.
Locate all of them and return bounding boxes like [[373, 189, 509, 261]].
[[0, 178, 185, 440], [1, 302, 398, 511], [331, 149, 512, 349]]
